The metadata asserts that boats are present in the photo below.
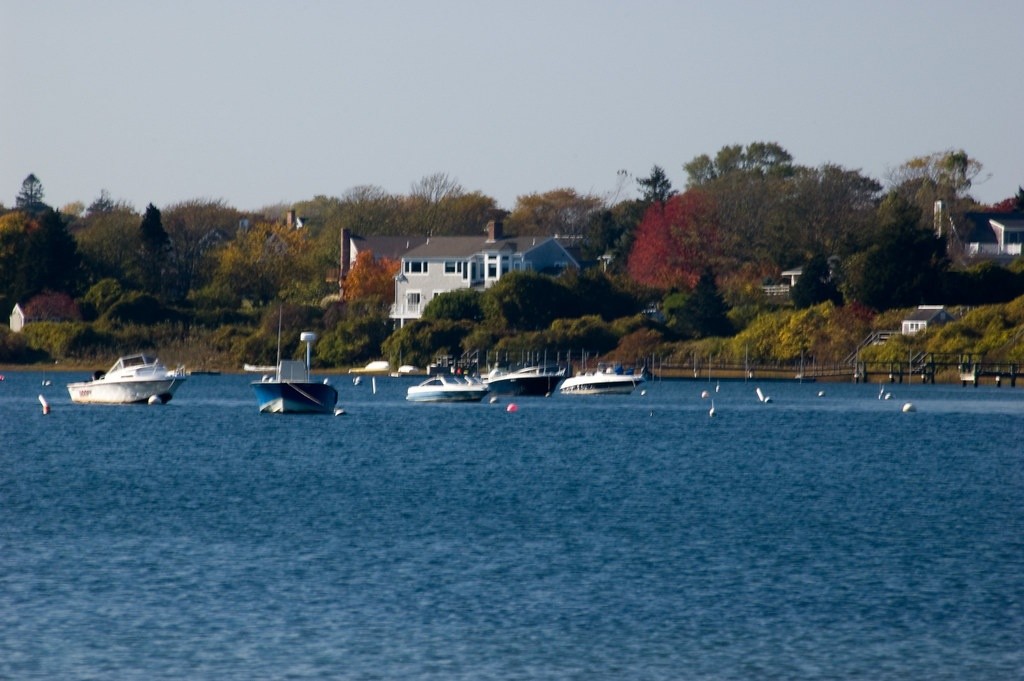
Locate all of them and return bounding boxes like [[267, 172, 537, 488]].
[[64, 352, 190, 404], [243, 361, 280, 372], [559, 364, 651, 394], [482, 365, 569, 397], [251, 303, 339, 414], [406, 371, 493, 402]]
